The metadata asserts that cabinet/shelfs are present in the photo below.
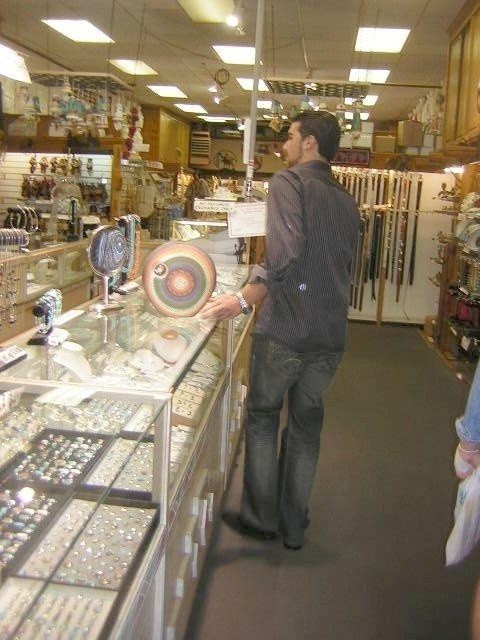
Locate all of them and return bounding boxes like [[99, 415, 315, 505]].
[[141, 104, 191, 172], [0, 262, 262, 640], [165, 177, 269, 262], [443, 0, 480, 160], [0, 231, 93, 343], [421, 161, 480, 387]]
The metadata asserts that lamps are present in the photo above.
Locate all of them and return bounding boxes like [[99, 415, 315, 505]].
[[225, 0, 243, 27], [301, 88, 312, 111], [270, 101, 278, 114]]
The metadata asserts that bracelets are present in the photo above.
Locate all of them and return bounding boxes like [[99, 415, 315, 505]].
[[458, 443, 480, 455], [36, 288, 62, 334]]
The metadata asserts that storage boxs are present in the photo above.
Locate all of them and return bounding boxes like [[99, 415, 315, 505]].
[[397, 120, 423, 147], [375, 136, 395, 153]]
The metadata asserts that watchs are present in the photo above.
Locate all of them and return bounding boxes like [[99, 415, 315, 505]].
[[236, 292, 253, 315]]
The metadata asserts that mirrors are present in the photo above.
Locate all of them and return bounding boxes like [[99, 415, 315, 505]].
[[88, 225, 127, 308]]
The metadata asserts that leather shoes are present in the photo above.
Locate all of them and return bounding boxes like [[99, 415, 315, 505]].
[[221, 512, 302, 551]]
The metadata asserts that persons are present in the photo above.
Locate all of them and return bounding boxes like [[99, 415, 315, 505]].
[[200, 111, 360, 550], [454, 356, 480, 640], [185, 172, 209, 200]]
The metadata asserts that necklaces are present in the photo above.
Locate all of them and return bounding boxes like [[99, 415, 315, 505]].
[[0, 229, 24, 328]]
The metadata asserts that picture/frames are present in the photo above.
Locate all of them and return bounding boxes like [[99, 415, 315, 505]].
[[329, 148, 370, 166]]
